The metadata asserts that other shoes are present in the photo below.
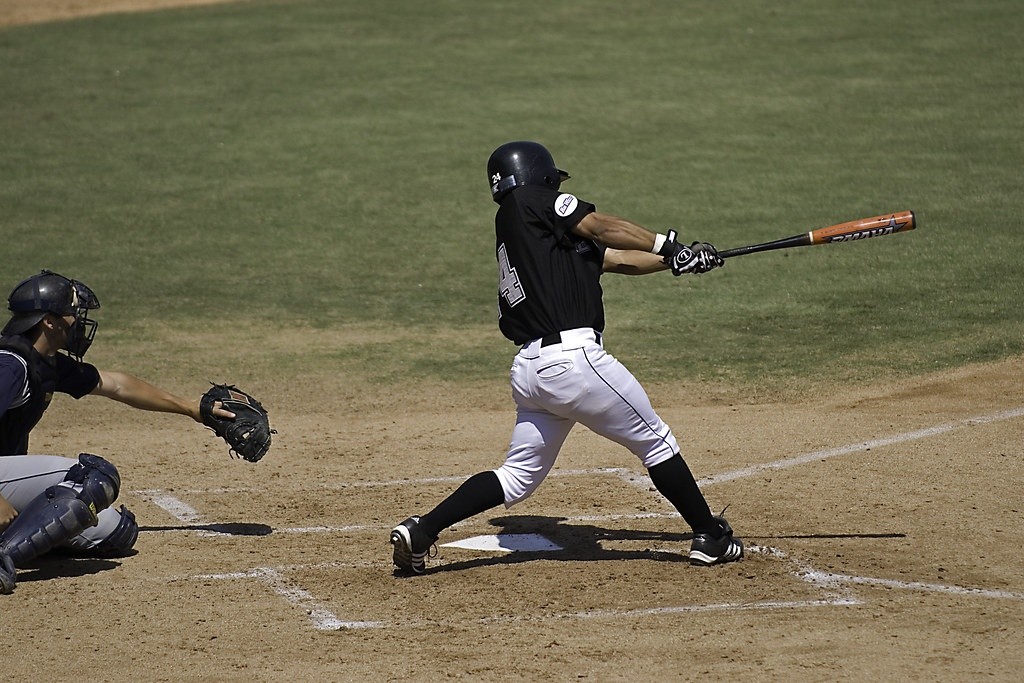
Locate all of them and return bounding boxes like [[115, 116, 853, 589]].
[[0, 566, 14, 595]]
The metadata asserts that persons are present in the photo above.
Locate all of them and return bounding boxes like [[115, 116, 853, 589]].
[[0, 270, 236, 594], [390, 142, 743, 573]]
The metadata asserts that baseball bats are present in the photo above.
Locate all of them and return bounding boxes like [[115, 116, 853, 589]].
[[672, 208, 917, 277]]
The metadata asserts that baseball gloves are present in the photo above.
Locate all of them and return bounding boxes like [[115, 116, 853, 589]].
[[199, 383, 271, 463]]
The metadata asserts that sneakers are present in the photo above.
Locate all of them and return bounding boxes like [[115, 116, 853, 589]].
[[689, 504, 744, 566], [390, 514, 439, 576]]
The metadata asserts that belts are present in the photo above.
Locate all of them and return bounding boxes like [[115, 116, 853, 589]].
[[541, 330, 600, 348]]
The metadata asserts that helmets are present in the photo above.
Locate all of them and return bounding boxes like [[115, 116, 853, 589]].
[[1, 268, 82, 335], [487, 141, 571, 205]]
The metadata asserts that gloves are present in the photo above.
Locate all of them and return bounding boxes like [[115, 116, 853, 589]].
[[652, 229, 699, 276], [687, 241, 725, 274]]
